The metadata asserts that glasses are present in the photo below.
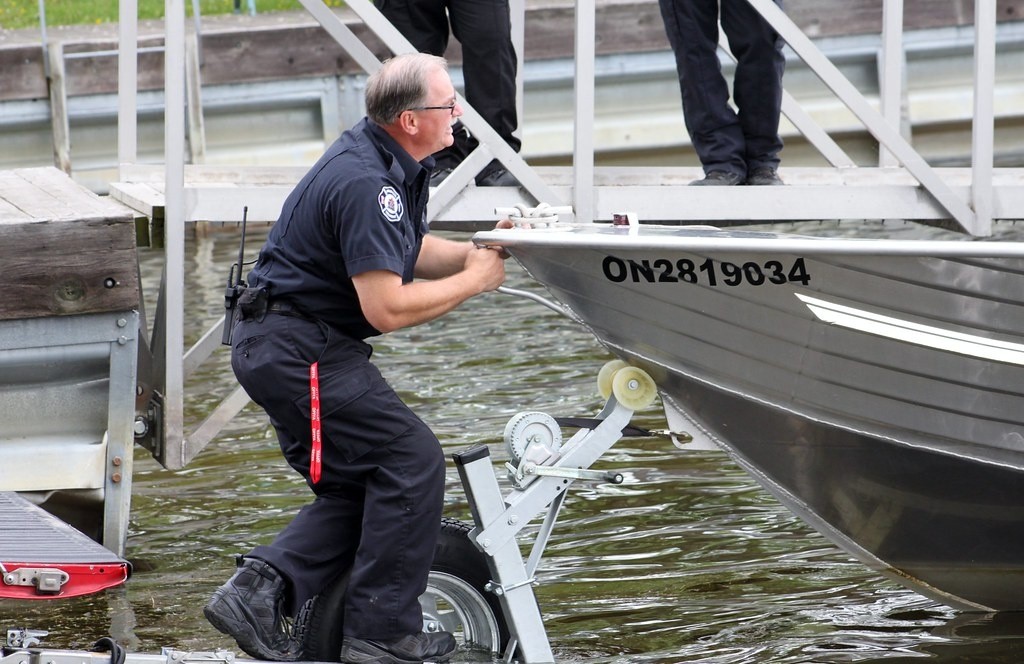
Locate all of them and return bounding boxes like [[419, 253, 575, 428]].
[[397, 97, 457, 116]]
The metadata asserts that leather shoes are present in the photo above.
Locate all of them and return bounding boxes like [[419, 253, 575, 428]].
[[203, 554, 304, 662], [341, 631, 457, 664]]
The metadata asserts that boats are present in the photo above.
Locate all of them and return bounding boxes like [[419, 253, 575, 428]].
[[468, 205, 1024, 617]]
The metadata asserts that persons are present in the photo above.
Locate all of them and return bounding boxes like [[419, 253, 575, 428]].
[[370, 0, 523, 187], [202, 48, 537, 664], [660, 1, 790, 186]]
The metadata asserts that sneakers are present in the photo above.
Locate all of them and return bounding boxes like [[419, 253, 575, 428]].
[[429, 165, 454, 186], [476, 168, 523, 186], [687, 167, 786, 185]]
